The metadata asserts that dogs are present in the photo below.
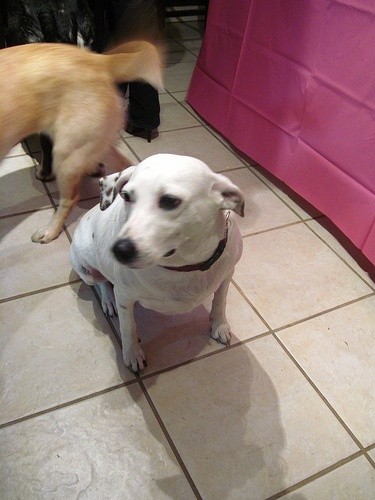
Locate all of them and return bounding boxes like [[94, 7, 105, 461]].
[[69, 153, 246, 374], [1, 0, 113, 184], [0, 42, 165, 245]]
